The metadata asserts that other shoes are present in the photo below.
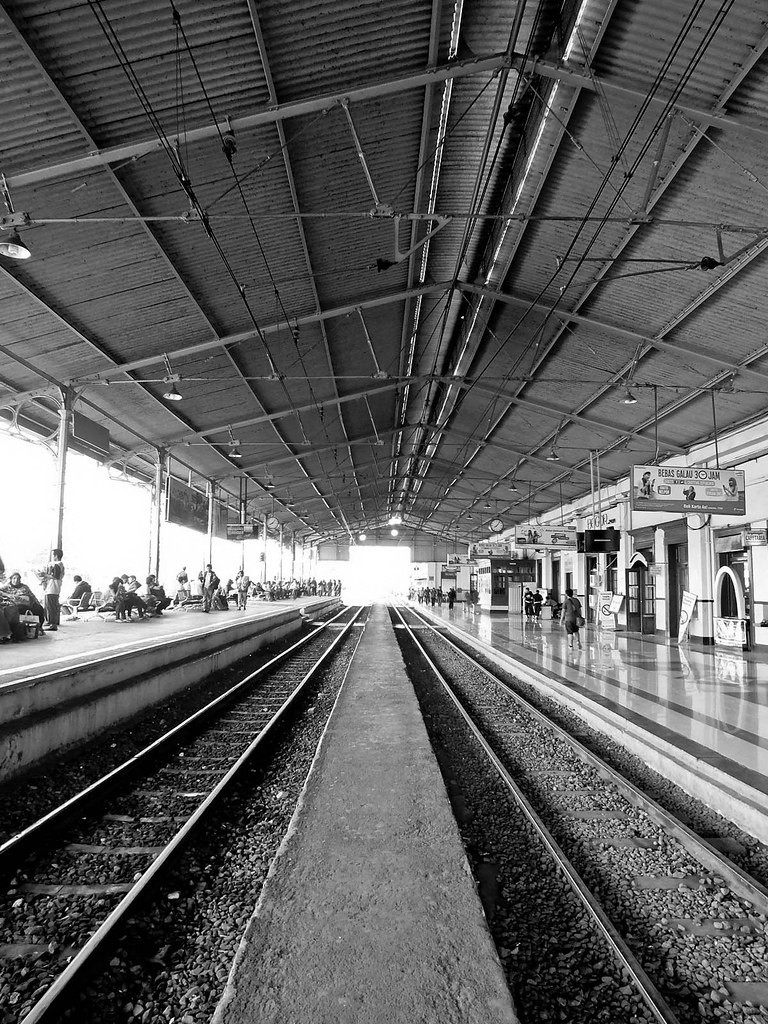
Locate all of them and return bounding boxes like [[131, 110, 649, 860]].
[[155, 609, 163, 615], [577, 641, 582, 650], [115, 619, 127, 623], [127, 616, 133, 621], [43, 626, 57, 631], [201, 609, 209, 614], [568, 645, 574, 651]]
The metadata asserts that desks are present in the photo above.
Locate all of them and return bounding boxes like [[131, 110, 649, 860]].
[[541, 607, 551, 620]]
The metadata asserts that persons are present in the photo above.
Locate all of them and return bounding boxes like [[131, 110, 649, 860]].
[[544, 596, 558, 619], [522, 587, 543, 621], [58, 576, 91, 613], [560, 589, 586, 650], [176, 564, 252, 613], [108, 575, 171, 624], [31, 550, 65, 632], [637, 471, 656, 500], [682, 485, 696, 501], [723, 477, 739, 502], [0, 556, 46, 644], [446, 586, 456, 612], [407, 586, 443, 607], [252, 576, 342, 602], [524, 529, 542, 544]]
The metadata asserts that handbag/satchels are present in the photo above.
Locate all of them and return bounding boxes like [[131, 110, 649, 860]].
[[211, 572, 220, 590], [19, 609, 39, 639], [576, 616, 585, 627]]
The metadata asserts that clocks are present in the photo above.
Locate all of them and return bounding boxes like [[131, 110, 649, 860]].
[[266, 518, 279, 530], [490, 519, 504, 532]]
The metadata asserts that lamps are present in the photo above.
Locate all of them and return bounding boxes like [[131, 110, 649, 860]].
[[483, 501, 491, 508], [546, 450, 559, 460], [617, 387, 637, 404], [304, 514, 309, 517], [466, 514, 473, 519], [287, 500, 294, 506], [163, 384, 182, 401], [228, 447, 241, 457], [265, 480, 275, 487], [508, 482, 517, 491]]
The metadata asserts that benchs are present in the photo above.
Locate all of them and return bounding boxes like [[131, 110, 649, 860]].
[[165, 590, 203, 612], [67, 591, 115, 623], [249, 589, 266, 601]]
